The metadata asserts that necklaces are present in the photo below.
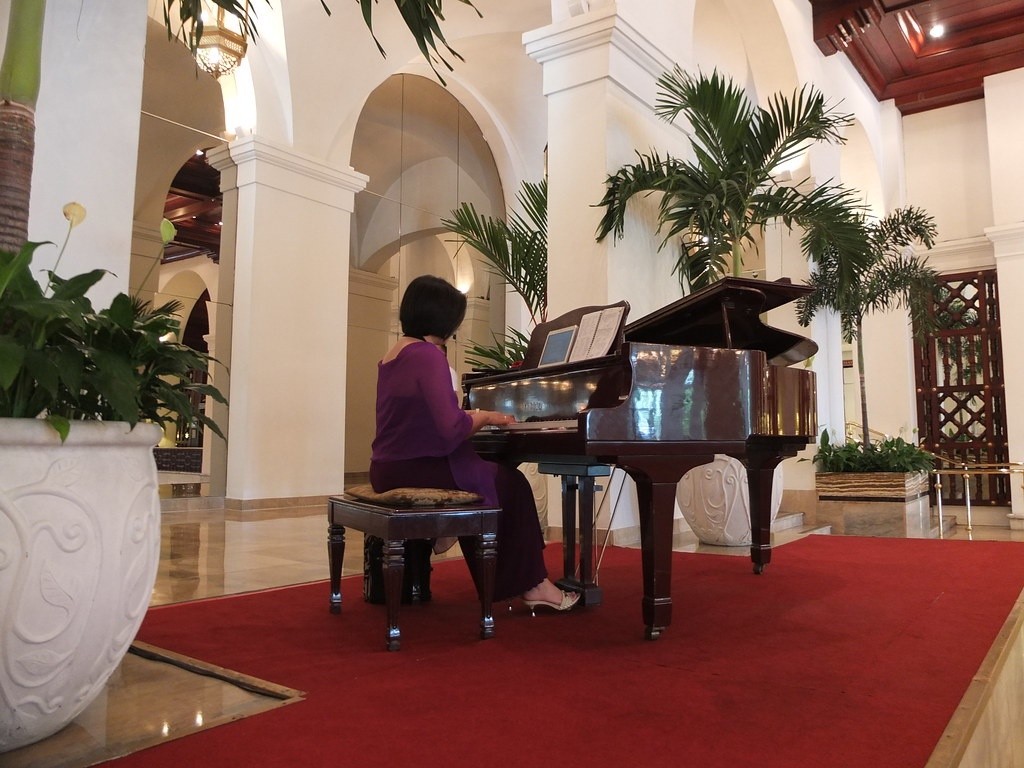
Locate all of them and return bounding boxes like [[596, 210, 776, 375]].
[[402, 333, 427, 342]]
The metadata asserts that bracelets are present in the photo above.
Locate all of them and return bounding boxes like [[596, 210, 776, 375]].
[[475, 408, 480, 414]]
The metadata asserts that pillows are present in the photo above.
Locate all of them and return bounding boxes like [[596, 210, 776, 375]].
[[342, 481, 485, 505]]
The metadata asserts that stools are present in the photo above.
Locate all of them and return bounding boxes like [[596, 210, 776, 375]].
[[325, 494, 505, 651]]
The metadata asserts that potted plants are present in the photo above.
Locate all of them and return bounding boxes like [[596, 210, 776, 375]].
[[797, 430, 939, 503], [1, 202, 230, 754], [438, 176, 548, 527], [590, 62, 882, 546]]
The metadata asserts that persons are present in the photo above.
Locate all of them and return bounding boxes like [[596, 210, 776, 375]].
[[369, 275, 582, 618]]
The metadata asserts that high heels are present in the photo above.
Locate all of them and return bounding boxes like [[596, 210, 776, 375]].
[[521, 588, 582, 617]]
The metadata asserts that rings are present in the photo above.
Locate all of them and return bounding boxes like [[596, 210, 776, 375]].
[[504, 416, 507, 420]]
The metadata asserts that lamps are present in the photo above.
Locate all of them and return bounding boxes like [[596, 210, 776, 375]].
[[191, 0, 250, 80]]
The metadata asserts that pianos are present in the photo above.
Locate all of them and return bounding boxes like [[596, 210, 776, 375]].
[[460, 274, 819, 643]]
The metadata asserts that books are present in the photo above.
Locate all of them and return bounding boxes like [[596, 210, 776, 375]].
[[569, 306, 626, 362]]
